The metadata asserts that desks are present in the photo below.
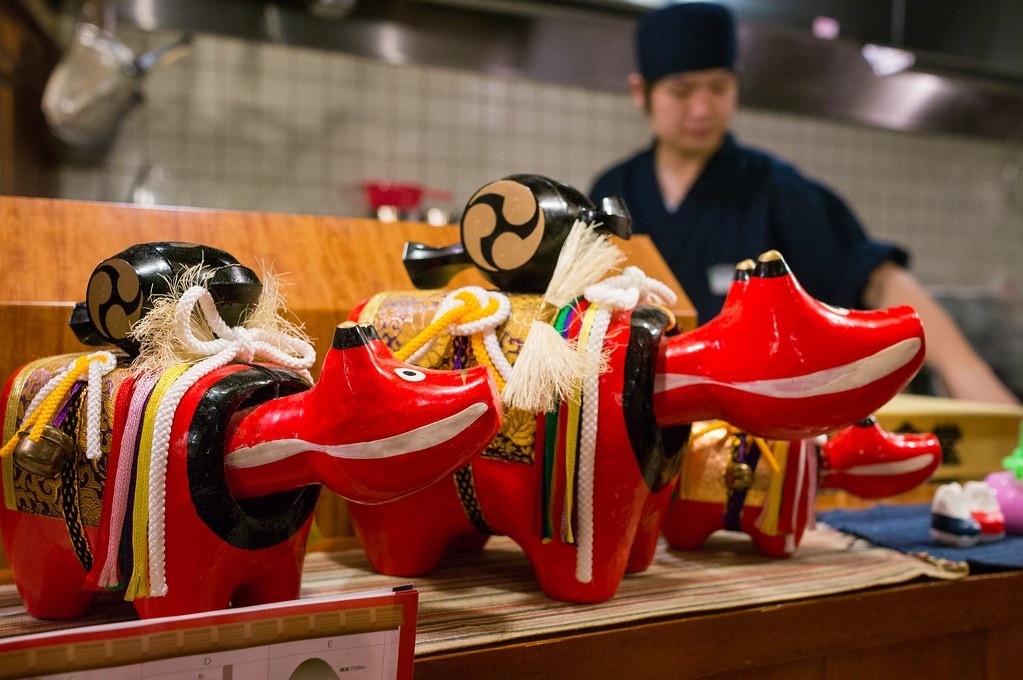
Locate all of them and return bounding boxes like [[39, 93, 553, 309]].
[[0, 530, 1023, 680]]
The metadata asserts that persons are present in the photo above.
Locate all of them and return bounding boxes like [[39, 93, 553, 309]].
[[590, 3, 1020, 406]]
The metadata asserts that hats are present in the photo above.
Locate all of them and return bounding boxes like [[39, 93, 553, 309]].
[[636, 2, 736, 76]]
